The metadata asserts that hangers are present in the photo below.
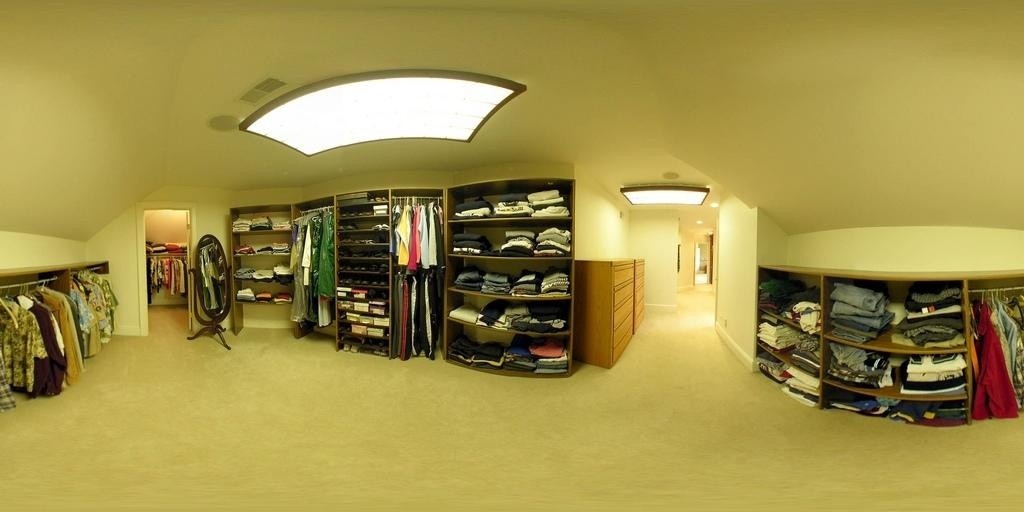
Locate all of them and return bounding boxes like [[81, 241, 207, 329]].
[[1, 267, 110, 330], [147, 256, 188, 264]]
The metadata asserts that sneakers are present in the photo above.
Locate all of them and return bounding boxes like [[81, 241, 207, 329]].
[[338, 311, 351, 333], [336, 195, 389, 299], [339, 334, 389, 356]]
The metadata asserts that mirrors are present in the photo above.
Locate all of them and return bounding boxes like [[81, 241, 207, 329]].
[[188, 234, 233, 350]]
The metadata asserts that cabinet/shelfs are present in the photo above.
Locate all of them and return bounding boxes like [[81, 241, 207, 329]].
[[230, 176, 576, 378], [754, 265, 1024, 426], [575, 258, 646, 370]]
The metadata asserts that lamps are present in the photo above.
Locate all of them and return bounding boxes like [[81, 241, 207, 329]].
[[620, 185, 711, 205], [239, 69, 527, 157]]
